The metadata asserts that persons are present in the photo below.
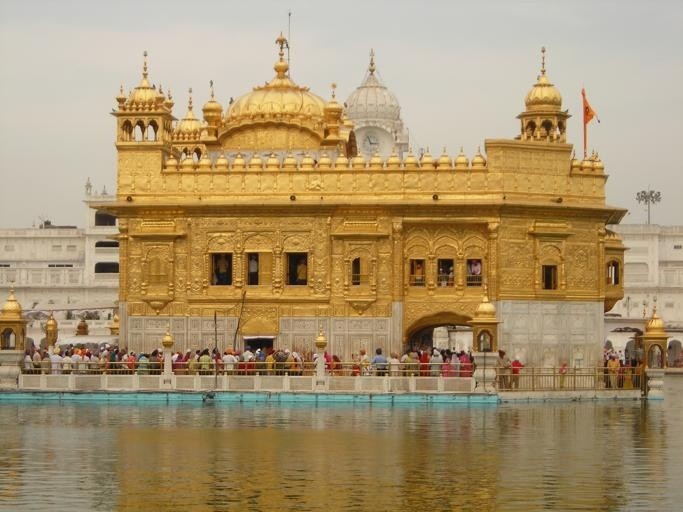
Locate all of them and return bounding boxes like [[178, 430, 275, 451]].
[[470, 259, 481, 286], [216, 254, 229, 282], [509, 356, 525, 390], [447, 265, 453, 285], [293, 256, 307, 285], [558, 360, 567, 390], [416, 262, 423, 281], [494, 350, 510, 390], [602, 348, 682, 388], [248, 255, 258, 281], [439, 267, 447, 287], [23, 341, 478, 377]]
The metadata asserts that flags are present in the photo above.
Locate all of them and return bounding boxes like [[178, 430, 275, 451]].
[[582, 88, 600, 128]]
